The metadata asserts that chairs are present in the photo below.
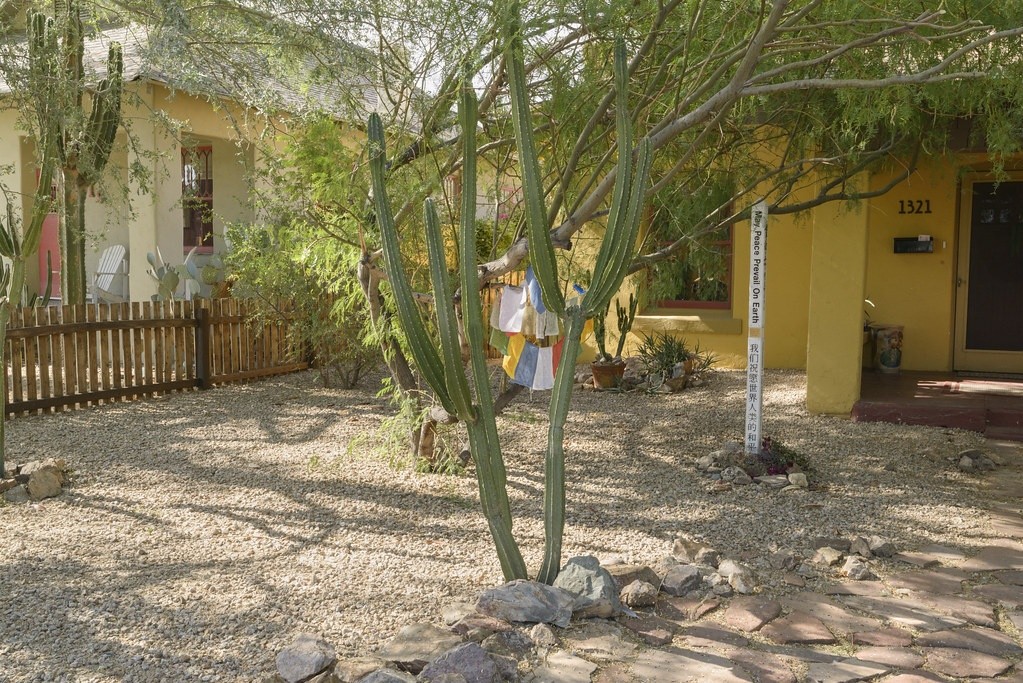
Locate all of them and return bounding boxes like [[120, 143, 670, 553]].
[[35, 245, 128, 304]]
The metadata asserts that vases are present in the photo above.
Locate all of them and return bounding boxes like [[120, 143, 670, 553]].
[[869, 323, 905, 373]]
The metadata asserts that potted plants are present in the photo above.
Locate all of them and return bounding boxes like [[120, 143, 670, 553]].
[[634, 327, 718, 391], [589, 268, 638, 389], [862, 292, 879, 345]]
